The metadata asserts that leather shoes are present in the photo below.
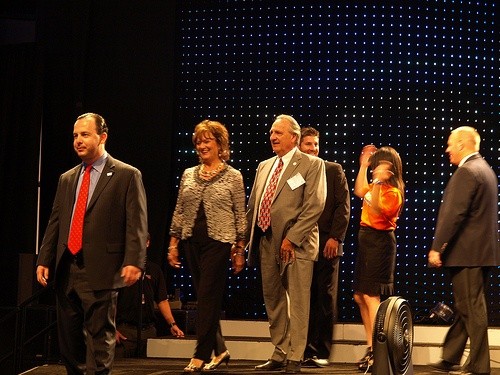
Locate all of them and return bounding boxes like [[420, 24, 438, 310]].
[[285, 361, 301, 374], [255, 360, 283, 371]]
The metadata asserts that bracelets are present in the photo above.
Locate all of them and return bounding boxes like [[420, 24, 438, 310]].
[[236, 245, 245, 248], [233, 252, 244, 256], [170, 323, 176, 327], [169, 246, 178, 250]]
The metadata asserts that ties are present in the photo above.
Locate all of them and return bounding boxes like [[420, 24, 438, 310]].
[[257, 157, 282, 231], [68, 166, 91, 255]]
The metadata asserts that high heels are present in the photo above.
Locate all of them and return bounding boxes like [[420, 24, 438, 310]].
[[184, 361, 204, 373], [205, 350, 230, 371]]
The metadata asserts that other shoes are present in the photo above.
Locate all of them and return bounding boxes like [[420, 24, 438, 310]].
[[427, 360, 459, 374]]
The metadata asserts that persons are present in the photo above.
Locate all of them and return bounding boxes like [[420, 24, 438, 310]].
[[354, 145, 404, 373], [36, 113, 147, 375], [428, 126, 497, 375], [299, 127, 350, 367], [230, 114, 327, 373], [116, 232, 184, 360], [169, 120, 248, 372]]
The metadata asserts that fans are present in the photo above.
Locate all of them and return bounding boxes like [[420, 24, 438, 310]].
[[370, 296, 414, 375]]
